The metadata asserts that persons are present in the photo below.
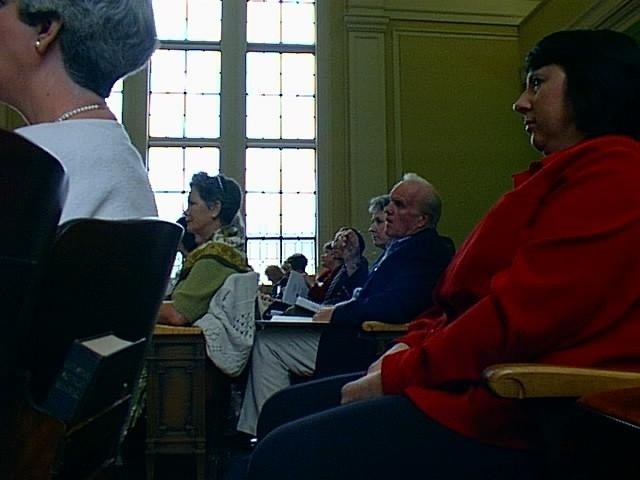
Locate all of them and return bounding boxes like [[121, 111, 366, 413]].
[[246, 25, 639, 480], [1, 1, 159, 373]]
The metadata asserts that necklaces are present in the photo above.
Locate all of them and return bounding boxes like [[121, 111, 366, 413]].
[[53, 104, 111, 122]]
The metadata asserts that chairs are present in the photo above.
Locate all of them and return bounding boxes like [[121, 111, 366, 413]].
[[477, 361, 640, 480], [362, 320, 413, 361], [116, 271, 261, 479], [1, 216, 186, 480]]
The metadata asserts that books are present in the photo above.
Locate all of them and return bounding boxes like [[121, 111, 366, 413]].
[[47, 328, 147, 427]]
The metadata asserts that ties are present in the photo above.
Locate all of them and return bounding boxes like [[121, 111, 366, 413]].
[[374, 239, 400, 274]]
